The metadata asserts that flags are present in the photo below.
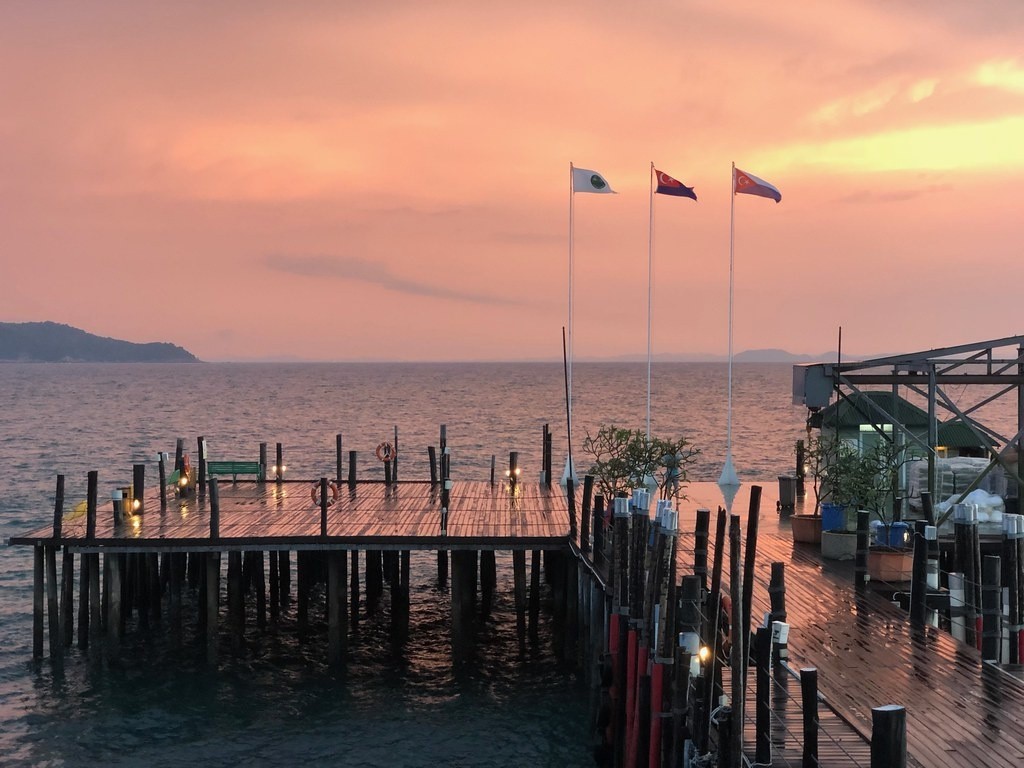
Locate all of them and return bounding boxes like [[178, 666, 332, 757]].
[[734, 167, 782, 205], [573, 168, 618, 194], [652, 167, 698, 200]]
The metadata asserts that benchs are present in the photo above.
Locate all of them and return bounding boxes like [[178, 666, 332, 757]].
[[207, 462, 261, 485]]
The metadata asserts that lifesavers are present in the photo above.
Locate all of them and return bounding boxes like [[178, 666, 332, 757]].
[[376, 441, 395, 462], [311, 481, 338, 508], [184, 453, 191, 475], [722, 594, 732, 661]]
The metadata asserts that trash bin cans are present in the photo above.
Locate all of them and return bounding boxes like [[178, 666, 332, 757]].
[[821, 501, 844, 530], [776, 475, 798, 510], [878, 521, 908, 550]]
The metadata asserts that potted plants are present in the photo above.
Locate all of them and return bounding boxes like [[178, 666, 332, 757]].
[[789, 434, 929, 580]]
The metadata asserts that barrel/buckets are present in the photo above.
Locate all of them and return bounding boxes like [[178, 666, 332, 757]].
[[876, 521, 908, 546], [820, 502, 845, 531]]
